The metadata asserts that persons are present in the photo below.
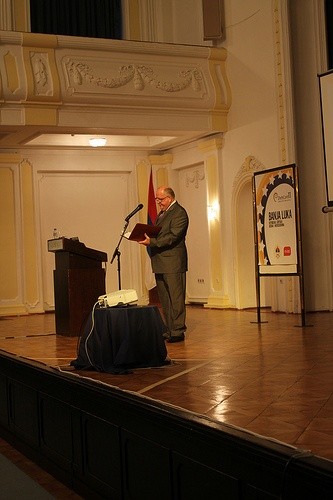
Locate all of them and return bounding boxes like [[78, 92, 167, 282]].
[[138, 186, 188, 343]]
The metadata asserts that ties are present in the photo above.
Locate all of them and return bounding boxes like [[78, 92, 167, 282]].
[[158, 210, 164, 220]]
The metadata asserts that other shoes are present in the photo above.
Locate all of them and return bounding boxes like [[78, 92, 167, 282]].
[[166, 335, 185, 343]]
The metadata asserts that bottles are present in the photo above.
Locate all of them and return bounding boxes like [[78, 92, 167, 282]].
[[53, 229, 58, 239]]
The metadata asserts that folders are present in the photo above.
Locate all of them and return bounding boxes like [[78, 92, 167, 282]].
[[120, 223, 163, 241]]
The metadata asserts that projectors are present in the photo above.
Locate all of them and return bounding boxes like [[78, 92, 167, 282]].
[[98, 289, 138, 308]]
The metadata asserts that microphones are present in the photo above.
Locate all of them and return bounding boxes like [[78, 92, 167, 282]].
[[125, 204, 143, 221]]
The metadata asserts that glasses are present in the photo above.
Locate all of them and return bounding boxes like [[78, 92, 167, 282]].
[[155, 196, 169, 201]]
